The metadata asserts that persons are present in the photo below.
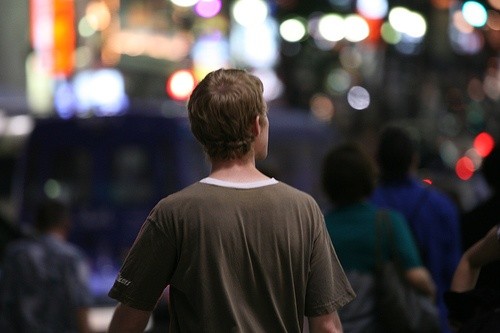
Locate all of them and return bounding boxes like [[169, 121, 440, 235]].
[[446, 220, 500, 333], [0, 194, 91, 333], [373, 122, 465, 333], [314, 146, 436, 333], [461, 147, 500, 248], [102, 67, 355, 333]]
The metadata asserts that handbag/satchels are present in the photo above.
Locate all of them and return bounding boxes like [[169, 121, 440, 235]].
[[373, 213, 425, 330]]
